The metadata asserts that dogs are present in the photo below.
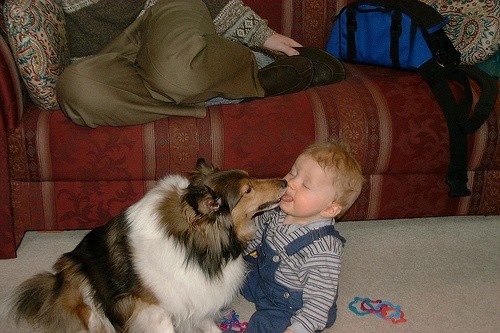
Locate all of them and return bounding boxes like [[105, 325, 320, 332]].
[[4, 159, 290, 333]]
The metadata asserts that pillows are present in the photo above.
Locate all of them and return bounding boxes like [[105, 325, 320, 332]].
[[424, 0, 500, 65], [3, 0, 67, 109]]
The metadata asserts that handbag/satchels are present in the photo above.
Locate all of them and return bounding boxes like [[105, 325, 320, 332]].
[[322, 0, 499, 198]]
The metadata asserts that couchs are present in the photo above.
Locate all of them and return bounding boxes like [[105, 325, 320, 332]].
[[0, 0, 500, 259]]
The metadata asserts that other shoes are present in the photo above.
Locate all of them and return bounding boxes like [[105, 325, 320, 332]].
[[230, 55, 315, 101], [278, 47, 346, 88]]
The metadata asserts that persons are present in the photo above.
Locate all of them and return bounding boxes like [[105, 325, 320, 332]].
[[53, 0, 346, 128], [227, 142, 365, 333]]
